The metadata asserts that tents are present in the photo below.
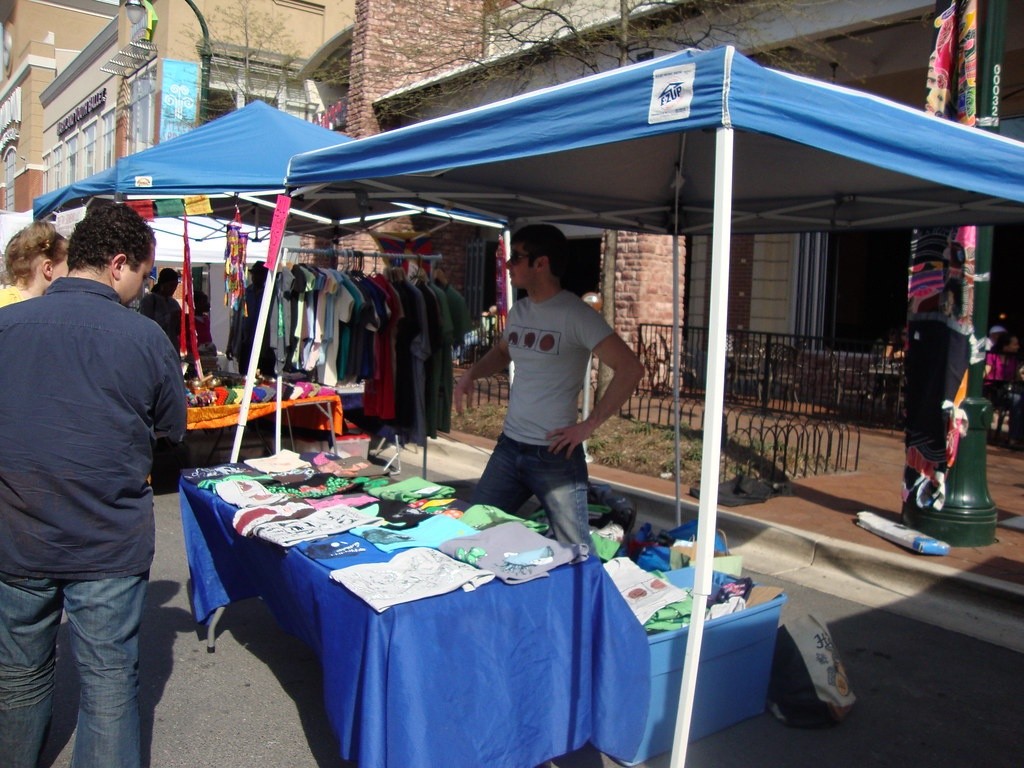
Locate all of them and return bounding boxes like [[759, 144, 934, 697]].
[[32, 100, 504, 454], [238, 44, 1024, 768]]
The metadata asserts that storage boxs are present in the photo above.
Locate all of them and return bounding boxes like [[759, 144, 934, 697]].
[[297, 432, 372, 459], [618, 566, 790, 767]]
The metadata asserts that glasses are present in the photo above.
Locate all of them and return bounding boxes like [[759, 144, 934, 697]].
[[511, 251, 537, 264]]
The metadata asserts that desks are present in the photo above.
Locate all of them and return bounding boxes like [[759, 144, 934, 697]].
[[870, 368, 904, 427], [184, 393, 343, 455], [337, 392, 402, 475], [180, 451, 602, 768]]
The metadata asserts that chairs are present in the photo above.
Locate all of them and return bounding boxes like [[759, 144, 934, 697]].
[[658, 334, 801, 409], [825, 348, 871, 418]]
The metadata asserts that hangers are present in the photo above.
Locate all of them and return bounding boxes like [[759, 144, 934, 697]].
[[286, 248, 448, 284], [229, 202, 245, 234]]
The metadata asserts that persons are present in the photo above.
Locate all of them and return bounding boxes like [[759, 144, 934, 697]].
[[983, 332, 1024, 449], [0, 203, 188, 768], [454, 224, 645, 560], [139, 268, 180, 358], [225, 260, 277, 376], [185, 290, 212, 346], [986, 325, 1006, 350], [0, 220, 69, 309]]
